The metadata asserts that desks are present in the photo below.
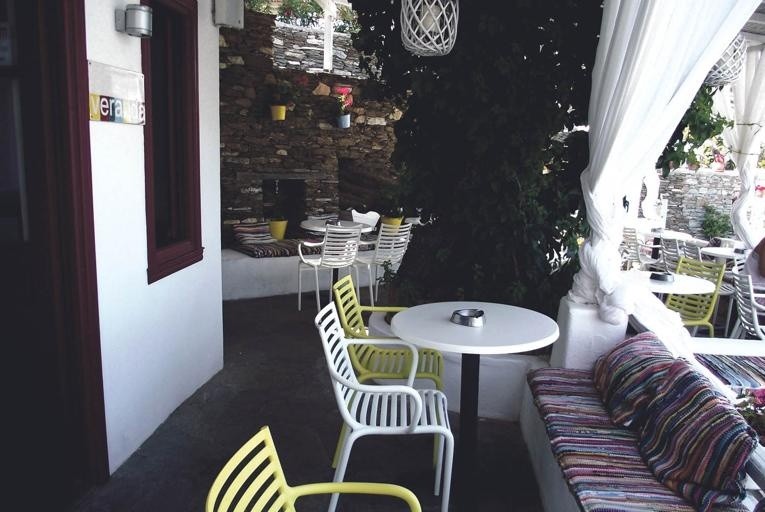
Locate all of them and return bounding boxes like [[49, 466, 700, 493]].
[[300, 218, 372, 300], [391, 301, 560, 481]]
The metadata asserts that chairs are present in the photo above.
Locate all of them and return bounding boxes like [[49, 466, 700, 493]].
[[622, 220, 765, 338], [299, 224, 361, 313], [330, 275, 444, 470], [314, 301, 454, 512], [357, 224, 413, 302], [205, 425, 422, 512]]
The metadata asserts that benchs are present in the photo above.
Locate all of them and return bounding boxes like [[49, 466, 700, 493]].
[[518, 330, 765, 512], [221, 239, 387, 301]]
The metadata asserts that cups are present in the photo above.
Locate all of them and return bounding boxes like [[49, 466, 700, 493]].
[[272, 105, 286, 121], [384, 218, 404, 237], [269, 220, 289, 240]]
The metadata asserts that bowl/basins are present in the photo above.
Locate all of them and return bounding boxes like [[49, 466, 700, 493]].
[[389, 207, 404, 218]]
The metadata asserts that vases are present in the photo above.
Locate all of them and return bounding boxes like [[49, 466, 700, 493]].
[[339, 114, 350, 128], [270, 105, 286, 121]]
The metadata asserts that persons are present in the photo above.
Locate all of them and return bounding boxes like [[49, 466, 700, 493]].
[[744, 238, 765, 326]]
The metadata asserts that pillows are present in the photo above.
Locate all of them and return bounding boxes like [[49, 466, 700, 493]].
[[233, 222, 274, 243], [630, 356, 758, 511], [593, 331, 677, 427]]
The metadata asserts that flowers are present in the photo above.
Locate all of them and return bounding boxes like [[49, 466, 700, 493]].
[[272, 73, 308, 113], [335, 85, 353, 116]]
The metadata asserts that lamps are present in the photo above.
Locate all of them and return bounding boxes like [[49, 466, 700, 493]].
[[703, 33, 746, 88], [401, 1, 459, 56]]
